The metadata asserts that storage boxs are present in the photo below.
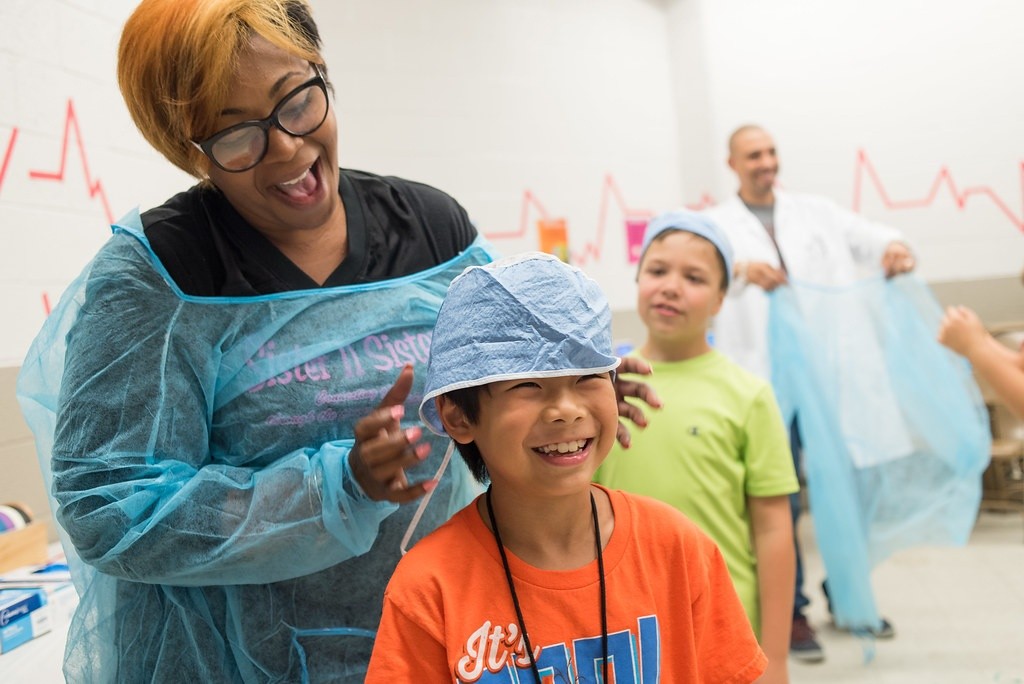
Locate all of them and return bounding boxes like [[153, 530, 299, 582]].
[[0, 586, 52, 653]]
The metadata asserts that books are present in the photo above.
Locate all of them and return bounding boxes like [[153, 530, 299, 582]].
[[0, 564, 81, 655]]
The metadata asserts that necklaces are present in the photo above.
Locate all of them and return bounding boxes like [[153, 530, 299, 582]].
[[486, 479, 609, 684]]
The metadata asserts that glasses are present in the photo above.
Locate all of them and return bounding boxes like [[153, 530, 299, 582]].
[[190, 60, 330, 174]]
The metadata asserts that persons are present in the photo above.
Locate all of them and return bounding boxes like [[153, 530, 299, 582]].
[[593, 210, 803, 684], [48, 1, 663, 683], [363, 252, 772, 684], [934, 307, 1024, 407], [692, 123, 917, 662]]
